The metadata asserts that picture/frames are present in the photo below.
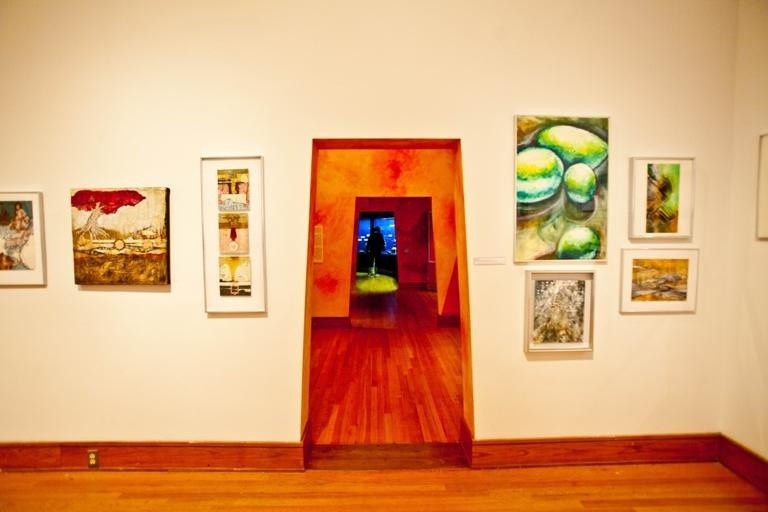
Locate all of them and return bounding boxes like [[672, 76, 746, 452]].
[[199, 154, 267, 316], [524, 266, 595, 354], [0, 191, 49, 288], [619, 154, 700, 315]]
